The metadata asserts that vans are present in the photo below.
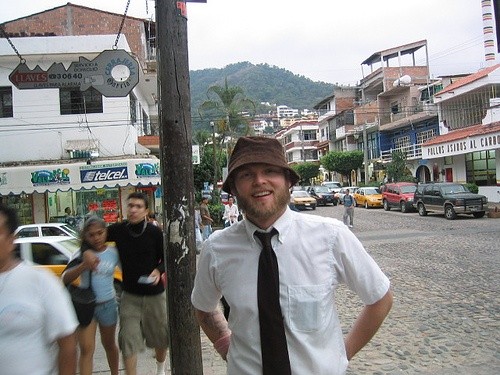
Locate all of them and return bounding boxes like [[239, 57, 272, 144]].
[[337, 187, 358, 204]]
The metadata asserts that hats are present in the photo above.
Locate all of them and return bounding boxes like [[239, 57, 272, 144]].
[[222, 136, 301, 196]]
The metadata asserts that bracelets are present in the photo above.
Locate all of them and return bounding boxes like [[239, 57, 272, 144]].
[[210, 333, 233, 355]]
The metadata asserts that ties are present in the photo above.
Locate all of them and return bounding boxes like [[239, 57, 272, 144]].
[[253, 228, 292, 375]]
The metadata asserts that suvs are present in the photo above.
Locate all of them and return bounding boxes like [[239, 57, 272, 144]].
[[413, 183, 487, 220], [382, 182, 418, 213], [321, 182, 342, 197]]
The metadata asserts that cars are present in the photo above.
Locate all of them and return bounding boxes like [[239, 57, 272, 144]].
[[201, 188, 236, 205], [12, 223, 79, 241], [13, 236, 123, 303], [292, 191, 317, 210], [354, 187, 384, 209], [310, 187, 338, 207]]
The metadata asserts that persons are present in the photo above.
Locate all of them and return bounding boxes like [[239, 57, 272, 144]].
[[0, 205, 81, 373], [64, 206, 79, 218], [221, 195, 239, 227], [108, 192, 171, 374], [59, 211, 122, 373], [200, 195, 214, 241], [342, 188, 357, 227], [195, 203, 205, 245], [188, 136, 395, 374]]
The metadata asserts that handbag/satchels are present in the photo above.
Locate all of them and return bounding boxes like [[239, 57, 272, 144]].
[[60, 257, 97, 328]]
